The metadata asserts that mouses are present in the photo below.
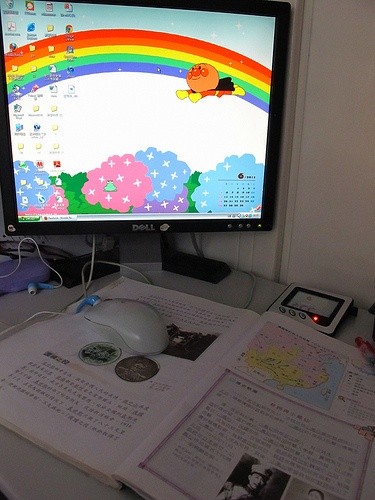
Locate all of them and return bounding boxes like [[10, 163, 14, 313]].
[[82, 297, 169, 356]]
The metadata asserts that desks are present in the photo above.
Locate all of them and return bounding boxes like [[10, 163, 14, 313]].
[[0, 254, 375, 499]]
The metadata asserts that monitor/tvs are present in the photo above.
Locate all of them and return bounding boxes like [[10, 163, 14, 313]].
[[0, 0, 291, 236]]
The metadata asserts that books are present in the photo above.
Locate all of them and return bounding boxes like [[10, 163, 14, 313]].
[[0, 275, 375, 500]]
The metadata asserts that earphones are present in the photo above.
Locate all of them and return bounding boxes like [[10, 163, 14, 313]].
[[76, 295, 103, 313], [27, 282, 54, 296]]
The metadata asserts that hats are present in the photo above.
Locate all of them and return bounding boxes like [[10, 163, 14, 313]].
[[251, 465, 272, 480]]
